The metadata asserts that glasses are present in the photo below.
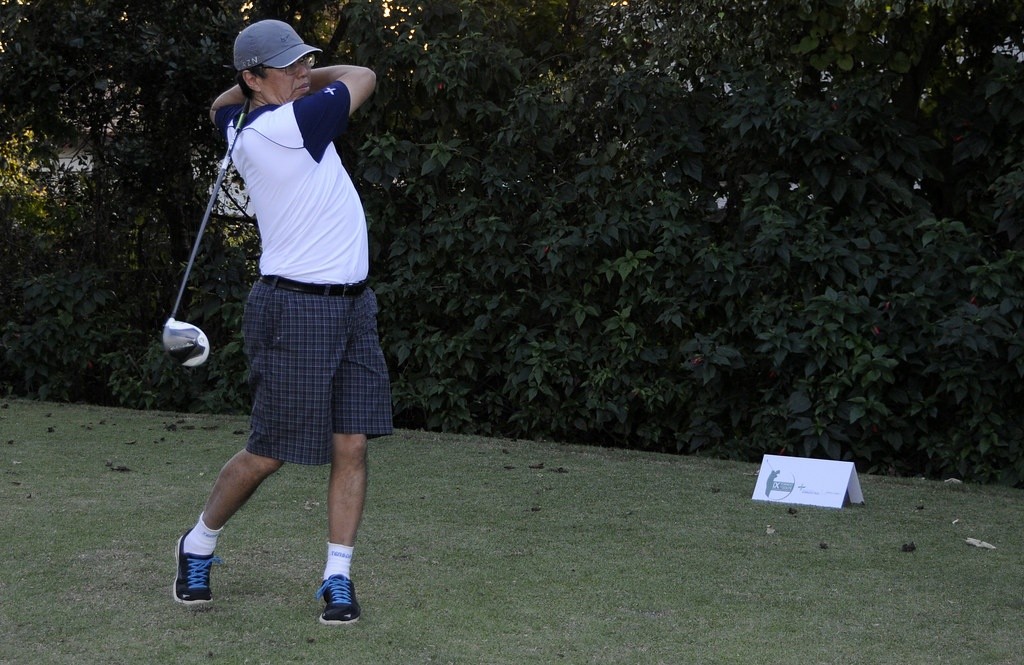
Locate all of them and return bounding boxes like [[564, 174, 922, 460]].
[[262, 54, 315, 75]]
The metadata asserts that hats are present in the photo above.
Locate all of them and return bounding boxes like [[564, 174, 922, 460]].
[[232, 20, 322, 69]]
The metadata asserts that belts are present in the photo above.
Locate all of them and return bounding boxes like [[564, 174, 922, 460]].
[[260, 275, 369, 301]]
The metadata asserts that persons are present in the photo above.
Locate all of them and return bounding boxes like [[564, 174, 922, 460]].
[[172, 20, 392, 627]]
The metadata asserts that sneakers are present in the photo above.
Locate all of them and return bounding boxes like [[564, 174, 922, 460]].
[[174, 529, 217, 605], [318, 573, 361, 625]]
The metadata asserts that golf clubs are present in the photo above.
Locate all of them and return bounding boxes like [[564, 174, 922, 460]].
[[160, 96, 252, 369]]
